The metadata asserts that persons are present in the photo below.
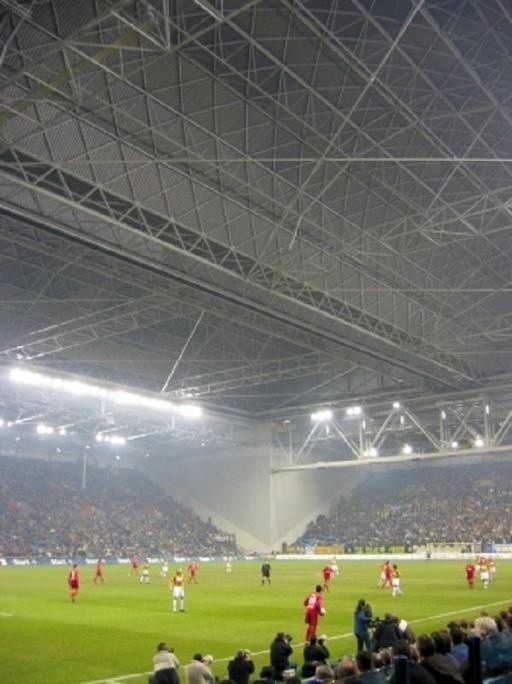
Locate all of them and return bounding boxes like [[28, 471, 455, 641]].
[[272, 457, 512, 554], [351, 598, 374, 654], [322, 557, 341, 593], [461, 554, 496, 589], [149, 612, 512, 684], [139, 557, 151, 584], [376, 560, 404, 599], [169, 568, 185, 613], [129, 555, 138, 577], [260, 559, 271, 587], [67, 564, 80, 602], [185, 558, 198, 585], [92, 558, 105, 585], [1, 455, 247, 557], [302, 584, 327, 647]]
